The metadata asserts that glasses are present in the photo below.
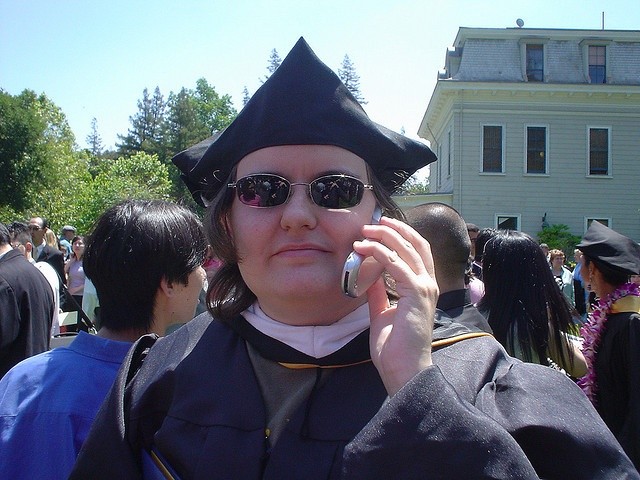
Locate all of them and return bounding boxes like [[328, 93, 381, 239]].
[[12, 243, 21, 250], [228, 174, 372, 209], [28, 226, 42, 231]]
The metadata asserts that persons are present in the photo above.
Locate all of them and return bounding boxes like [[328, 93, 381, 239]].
[[236, 175, 290, 207], [311, 176, 364, 208], [64, 235, 86, 308], [0, 199, 207, 480], [59, 225, 77, 259], [571, 248, 589, 303], [0, 221, 54, 382], [44, 228, 59, 249], [575, 219, 640, 474], [472, 228, 499, 280], [549, 249, 576, 307], [466, 224, 479, 257], [28, 216, 67, 292], [67, 36, 640, 480], [403, 202, 494, 335], [7, 221, 60, 338], [539, 243, 551, 262], [474, 230, 588, 379]]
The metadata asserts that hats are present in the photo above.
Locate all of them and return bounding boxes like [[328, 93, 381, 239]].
[[576, 220, 640, 276], [171, 37, 438, 209]]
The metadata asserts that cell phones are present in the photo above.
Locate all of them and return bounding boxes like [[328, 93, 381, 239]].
[[339, 205, 382, 298]]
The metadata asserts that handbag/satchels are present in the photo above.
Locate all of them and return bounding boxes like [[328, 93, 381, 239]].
[[49, 327, 98, 350]]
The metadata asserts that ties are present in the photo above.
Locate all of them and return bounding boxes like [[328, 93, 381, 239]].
[[33, 247, 38, 261]]
